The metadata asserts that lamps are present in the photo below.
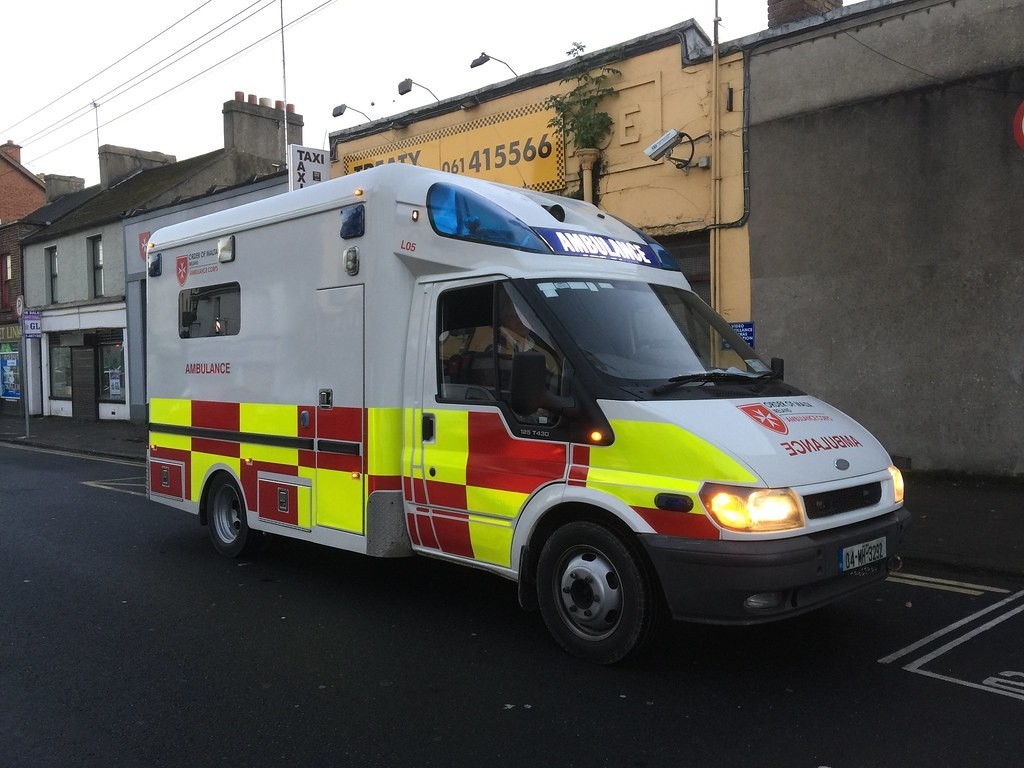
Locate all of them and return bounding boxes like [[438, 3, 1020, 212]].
[[469, 52, 518, 76], [332, 104, 372, 124], [398, 77, 439, 101]]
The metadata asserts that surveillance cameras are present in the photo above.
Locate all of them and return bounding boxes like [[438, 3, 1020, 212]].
[[643, 129, 682, 161]]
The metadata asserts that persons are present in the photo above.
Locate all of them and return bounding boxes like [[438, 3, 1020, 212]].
[[477, 299, 559, 424]]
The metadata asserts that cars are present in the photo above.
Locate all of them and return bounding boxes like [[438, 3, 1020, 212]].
[[55, 370, 66, 389]]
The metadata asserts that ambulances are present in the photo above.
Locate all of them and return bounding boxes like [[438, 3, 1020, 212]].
[[145, 163, 914, 666]]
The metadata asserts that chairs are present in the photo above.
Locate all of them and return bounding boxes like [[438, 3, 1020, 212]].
[[507, 348, 560, 425]]
